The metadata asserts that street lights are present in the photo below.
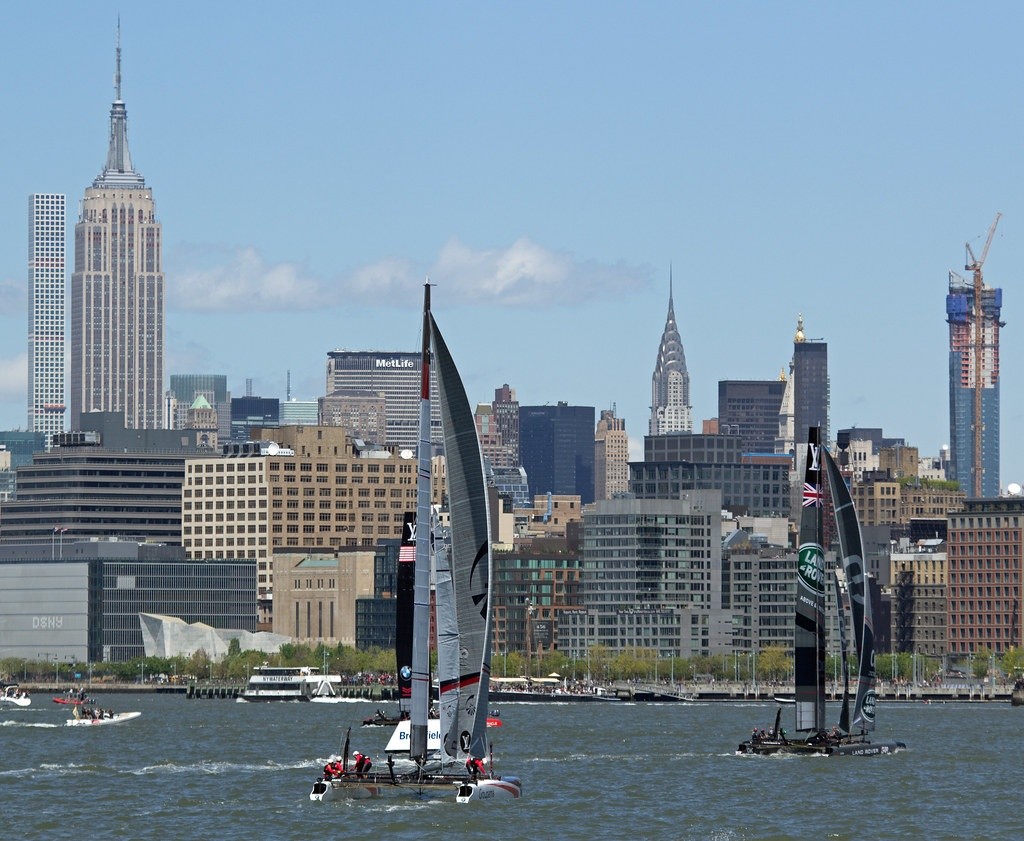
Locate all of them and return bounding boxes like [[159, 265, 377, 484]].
[[667, 653, 674, 688], [655, 652, 662, 685], [206, 663, 212, 683], [85, 660, 96, 688], [137, 660, 147, 685]]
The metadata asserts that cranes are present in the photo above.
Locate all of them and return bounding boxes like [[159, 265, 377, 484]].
[[964, 210, 1006, 497]]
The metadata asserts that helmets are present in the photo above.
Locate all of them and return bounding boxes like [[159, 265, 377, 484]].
[[353, 751, 359, 756], [328, 759, 334, 763], [336, 756, 342, 762], [482, 758, 488, 765]]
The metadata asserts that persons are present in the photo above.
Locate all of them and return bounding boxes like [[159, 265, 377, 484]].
[[350, 750, 372, 780], [341, 670, 398, 686], [489, 683, 585, 694], [832, 727, 841, 736], [768, 727, 774, 736], [466, 757, 487, 782], [323, 755, 346, 781], [758, 729, 766, 738], [751, 728, 758, 740]]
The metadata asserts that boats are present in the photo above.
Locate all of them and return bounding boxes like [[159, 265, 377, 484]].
[[0, 680, 32, 710], [235, 660, 343, 704], [51, 695, 90, 705], [64, 711, 143, 727]]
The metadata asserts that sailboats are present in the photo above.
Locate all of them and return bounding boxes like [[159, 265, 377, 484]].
[[738, 419, 907, 758], [309, 278, 522, 808]]
